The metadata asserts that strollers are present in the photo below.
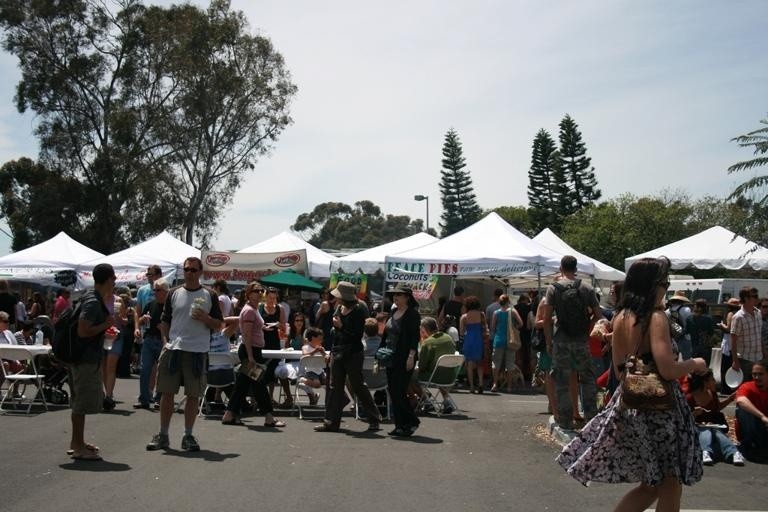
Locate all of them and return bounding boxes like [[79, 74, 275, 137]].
[[27, 327, 115, 412]]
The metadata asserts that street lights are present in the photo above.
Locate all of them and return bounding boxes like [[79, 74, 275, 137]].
[[415, 195, 429, 234]]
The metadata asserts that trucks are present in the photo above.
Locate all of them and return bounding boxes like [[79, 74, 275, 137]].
[[662, 276, 767, 323]]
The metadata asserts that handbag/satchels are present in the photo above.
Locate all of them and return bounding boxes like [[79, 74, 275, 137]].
[[507, 324, 522, 351], [621, 356, 678, 411], [375, 346, 396, 368], [530, 328, 546, 353]]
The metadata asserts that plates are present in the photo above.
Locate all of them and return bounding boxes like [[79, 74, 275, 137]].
[[698, 425, 724, 429], [724, 367, 743, 388]]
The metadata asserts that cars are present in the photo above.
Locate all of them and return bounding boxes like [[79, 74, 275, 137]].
[[356, 285, 391, 315]]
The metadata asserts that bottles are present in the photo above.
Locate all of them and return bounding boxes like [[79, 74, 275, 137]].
[[103, 327, 114, 351], [188, 297, 205, 319], [36, 329, 43, 345]]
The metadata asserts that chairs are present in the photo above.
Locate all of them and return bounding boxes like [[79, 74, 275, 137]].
[[1, 342, 71, 414], [172, 336, 392, 422], [412, 354, 466, 418]]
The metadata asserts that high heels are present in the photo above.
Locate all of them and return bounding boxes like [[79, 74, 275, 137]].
[[470, 386, 475, 393], [478, 384, 484, 394]]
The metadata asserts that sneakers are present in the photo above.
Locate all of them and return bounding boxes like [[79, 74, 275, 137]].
[[313, 419, 339, 431], [181, 434, 200, 452], [732, 451, 745, 465], [701, 451, 713, 465], [442, 400, 454, 414], [275, 392, 320, 407], [145, 433, 170, 450], [387, 420, 421, 438], [101, 391, 162, 411], [422, 400, 436, 414], [368, 414, 383, 431]]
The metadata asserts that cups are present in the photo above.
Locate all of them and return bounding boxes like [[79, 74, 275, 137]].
[[145, 315, 150, 329]]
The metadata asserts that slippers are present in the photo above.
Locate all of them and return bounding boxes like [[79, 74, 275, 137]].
[[68, 443, 101, 460], [263, 419, 286, 427], [221, 416, 244, 426]]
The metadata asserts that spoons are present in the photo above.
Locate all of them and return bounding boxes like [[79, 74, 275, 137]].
[[703, 408, 711, 412]]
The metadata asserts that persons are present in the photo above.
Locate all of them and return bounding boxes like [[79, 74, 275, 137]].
[[146, 254, 222, 451], [64, 259, 119, 460], [554, 254, 709, 511], [543, 254, 602, 423]]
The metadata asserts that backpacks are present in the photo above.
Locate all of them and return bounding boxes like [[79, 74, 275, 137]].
[[552, 278, 591, 340], [51, 292, 106, 366], [668, 305, 685, 339]]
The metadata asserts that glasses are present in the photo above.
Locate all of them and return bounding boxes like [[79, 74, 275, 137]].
[[251, 288, 265, 293], [183, 267, 201, 273]]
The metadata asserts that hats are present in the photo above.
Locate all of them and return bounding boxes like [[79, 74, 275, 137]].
[[385, 284, 413, 296], [669, 290, 690, 304], [723, 298, 741, 307], [328, 280, 357, 301]]
